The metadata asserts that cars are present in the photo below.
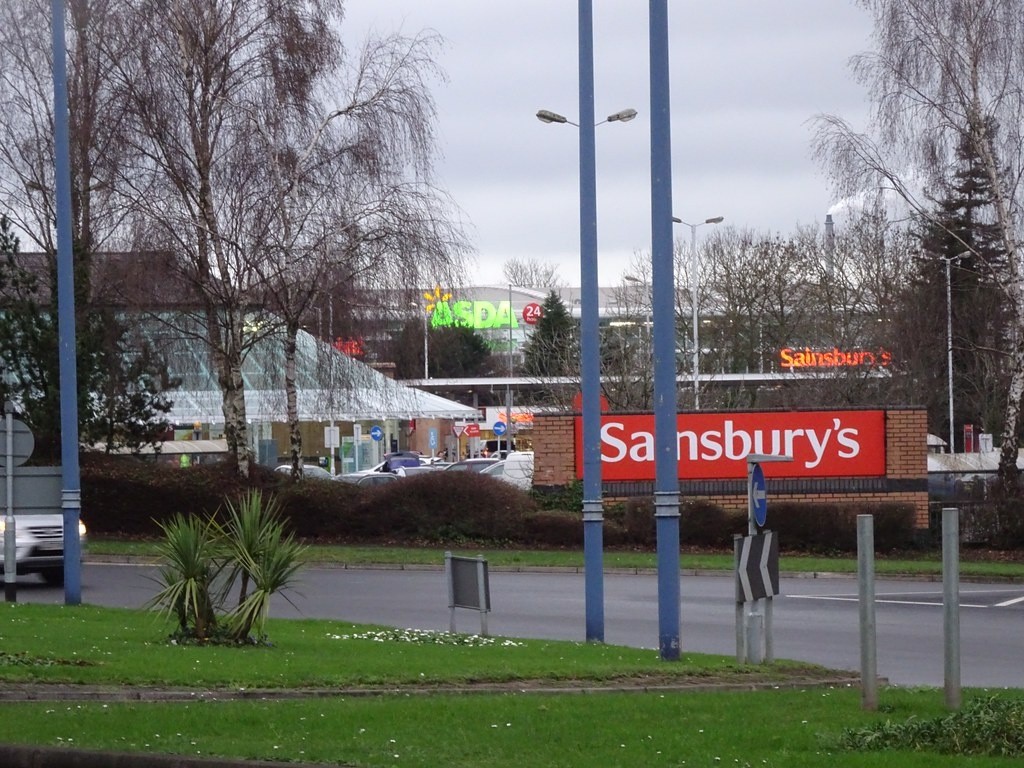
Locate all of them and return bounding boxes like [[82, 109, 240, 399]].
[[275, 448, 534, 493], [0, 513, 87, 587]]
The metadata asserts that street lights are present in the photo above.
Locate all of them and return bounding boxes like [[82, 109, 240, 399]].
[[673, 214, 725, 410], [936, 250, 972, 455]]
